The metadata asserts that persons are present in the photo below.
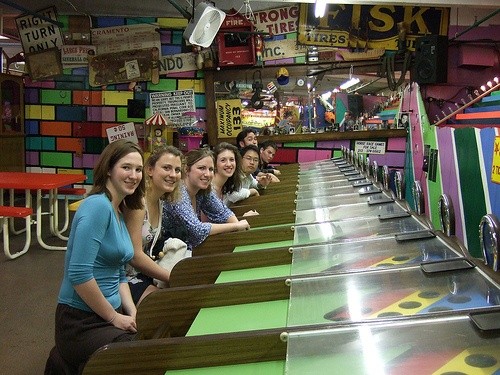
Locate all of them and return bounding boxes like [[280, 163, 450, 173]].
[[55, 140, 146, 371], [151, 149, 250, 256], [200, 142, 260, 224], [398, 114, 409, 128], [223, 145, 272, 207], [232, 129, 282, 182], [339, 110, 355, 132], [117, 145, 185, 316]]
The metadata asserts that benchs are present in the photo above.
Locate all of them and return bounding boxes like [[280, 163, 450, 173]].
[[0, 188, 92, 228], [0, 206, 33, 255]]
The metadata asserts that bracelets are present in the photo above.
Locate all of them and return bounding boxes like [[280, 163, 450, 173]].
[[106, 313, 118, 324]]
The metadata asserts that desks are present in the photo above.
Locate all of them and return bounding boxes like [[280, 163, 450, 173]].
[[0, 172, 86, 249]]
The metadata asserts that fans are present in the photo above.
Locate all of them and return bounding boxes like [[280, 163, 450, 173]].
[[168, 0, 227, 48]]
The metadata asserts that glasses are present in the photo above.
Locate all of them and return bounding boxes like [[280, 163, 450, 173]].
[[243, 156, 259, 162]]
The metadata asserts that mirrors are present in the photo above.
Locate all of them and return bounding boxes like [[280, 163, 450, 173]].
[[203, 64, 407, 146]]
